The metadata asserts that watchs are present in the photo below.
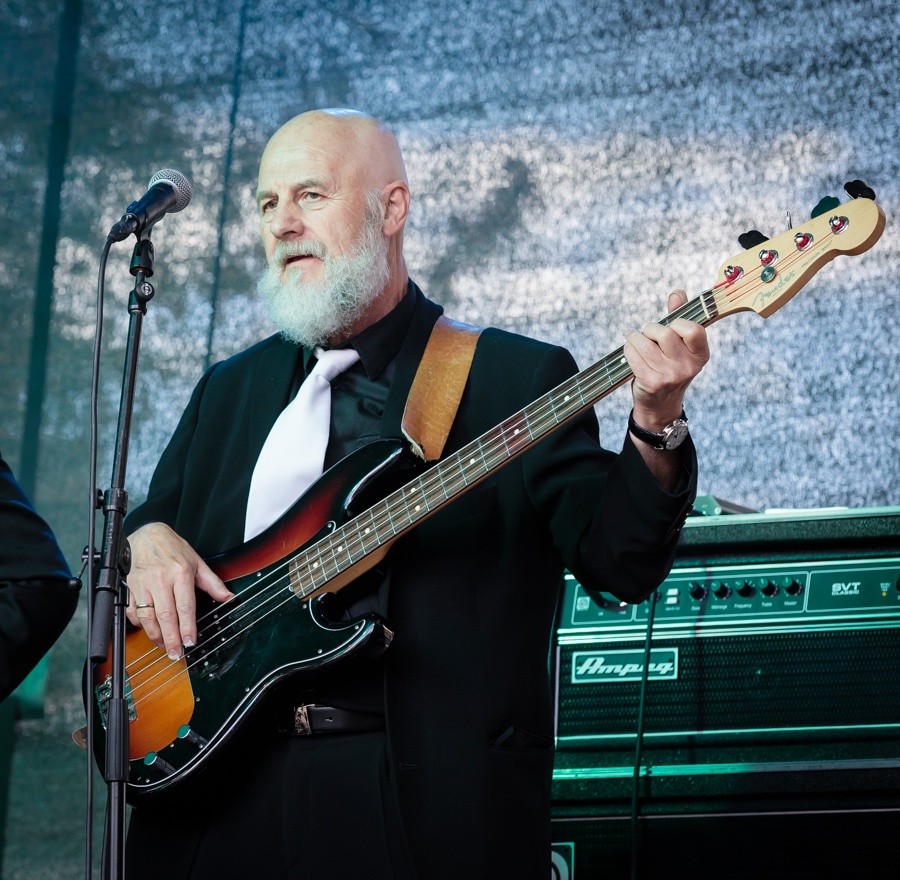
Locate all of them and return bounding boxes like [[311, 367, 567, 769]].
[[628, 404, 689, 451]]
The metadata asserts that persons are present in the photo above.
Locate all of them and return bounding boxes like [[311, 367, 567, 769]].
[[0, 453, 81, 703], [124, 108, 710, 880]]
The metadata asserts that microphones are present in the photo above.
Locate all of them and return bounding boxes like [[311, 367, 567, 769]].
[[106, 168, 192, 243]]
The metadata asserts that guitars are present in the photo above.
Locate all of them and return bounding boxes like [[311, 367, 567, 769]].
[[82, 179, 886, 798]]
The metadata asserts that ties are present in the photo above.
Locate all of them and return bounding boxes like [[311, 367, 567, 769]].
[[242, 346, 360, 544]]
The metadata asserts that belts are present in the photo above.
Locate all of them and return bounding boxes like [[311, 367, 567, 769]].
[[237, 705, 385, 738]]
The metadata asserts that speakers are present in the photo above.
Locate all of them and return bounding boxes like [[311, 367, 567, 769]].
[[553, 774, 900, 880]]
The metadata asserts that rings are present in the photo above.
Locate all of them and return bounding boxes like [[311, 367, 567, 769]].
[[136, 603, 154, 609]]
